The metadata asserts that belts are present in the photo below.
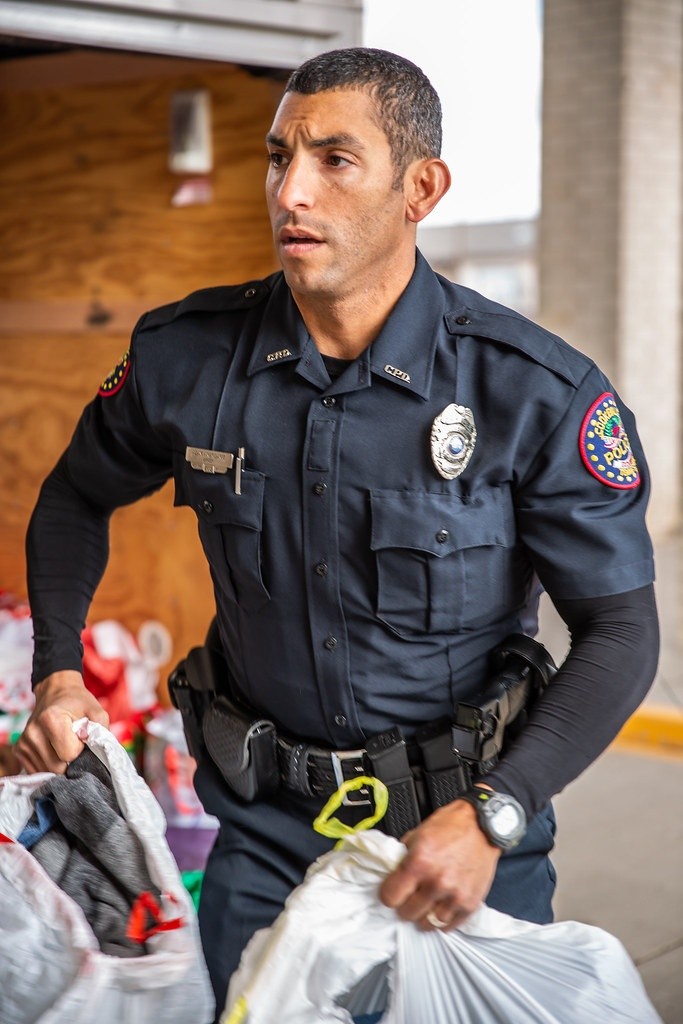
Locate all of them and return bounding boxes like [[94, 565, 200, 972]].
[[274, 711, 425, 806]]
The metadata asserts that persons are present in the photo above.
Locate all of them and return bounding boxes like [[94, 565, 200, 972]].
[[12, 47, 660, 1023]]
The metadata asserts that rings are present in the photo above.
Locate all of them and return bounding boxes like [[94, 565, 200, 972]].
[[427, 910, 452, 930]]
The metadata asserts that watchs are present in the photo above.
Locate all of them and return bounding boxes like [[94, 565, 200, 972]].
[[455, 785, 527, 854]]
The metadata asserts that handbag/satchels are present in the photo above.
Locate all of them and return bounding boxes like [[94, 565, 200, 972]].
[[0, 715, 219, 1024], [215, 776, 666, 1024]]
